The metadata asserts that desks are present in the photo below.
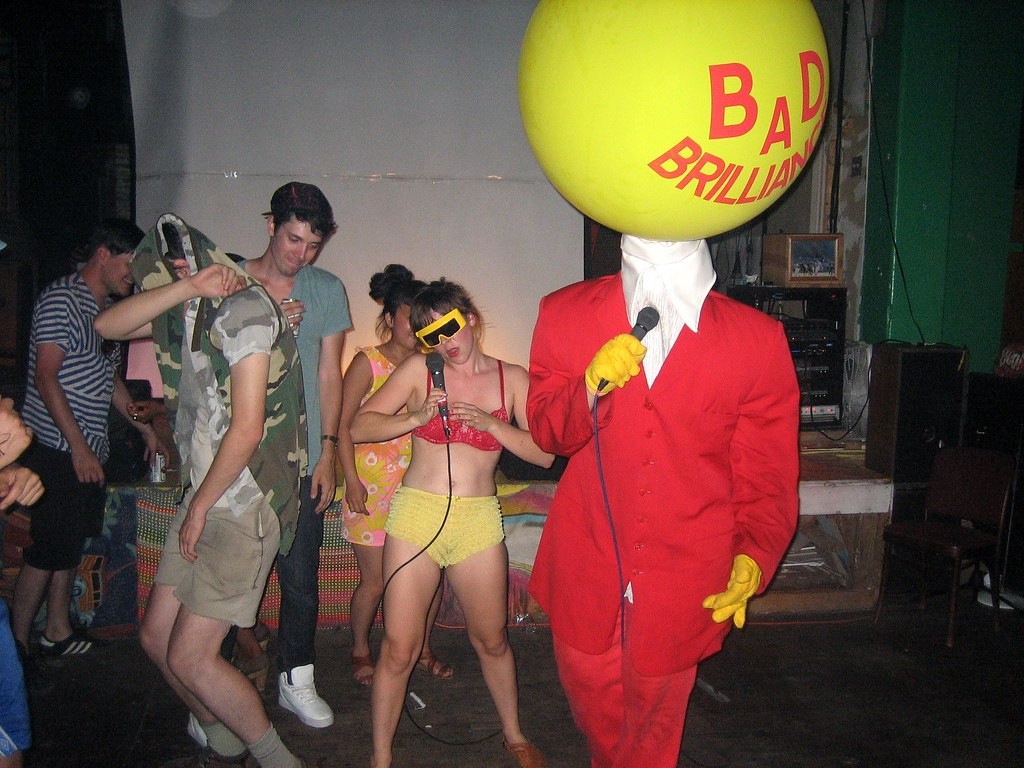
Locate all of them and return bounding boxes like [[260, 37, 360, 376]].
[[95, 481, 557, 646]]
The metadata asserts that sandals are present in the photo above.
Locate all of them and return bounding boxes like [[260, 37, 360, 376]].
[[348, 650, 373, 687], [416, 650, 455, 680]]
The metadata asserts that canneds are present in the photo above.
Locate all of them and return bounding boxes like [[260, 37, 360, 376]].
[[281, 298, 300, 337], [150, 453, 166, 482]]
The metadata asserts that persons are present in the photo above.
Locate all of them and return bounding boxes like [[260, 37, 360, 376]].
[[0, 218, 278, 768], [793, 258, 833, 277], [93, 213, 308, 768], [187, 181, 353, 747], [350, 278, 556, 768], [336, 264, 456, 690], [526, 237, 801, 768]]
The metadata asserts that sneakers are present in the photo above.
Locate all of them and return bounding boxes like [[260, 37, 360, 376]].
[[279, 663, 335, 727], [186, 712, 208, 747], [39, 629, 112, 661]]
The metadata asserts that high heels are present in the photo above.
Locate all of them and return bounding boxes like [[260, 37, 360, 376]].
[[253, 621, 271, 651], [233, 653, 271, 693]]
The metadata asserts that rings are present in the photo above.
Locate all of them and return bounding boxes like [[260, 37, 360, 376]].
[[133, 413, 137, 419]]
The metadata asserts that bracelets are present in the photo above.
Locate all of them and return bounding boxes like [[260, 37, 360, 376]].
[[321, 434, 339, 448]]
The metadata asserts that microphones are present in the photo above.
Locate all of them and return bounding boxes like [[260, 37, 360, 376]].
[[597, 306, 660, 391], [425, 351, 451, 436]]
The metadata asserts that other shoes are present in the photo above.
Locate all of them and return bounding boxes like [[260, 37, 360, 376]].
[[502, 734, 548, 768], [188, 745, 262, 768]]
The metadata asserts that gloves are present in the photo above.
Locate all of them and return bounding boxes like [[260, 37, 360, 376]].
[[583, 333, 647, 397], [702, 555, 761, 629]]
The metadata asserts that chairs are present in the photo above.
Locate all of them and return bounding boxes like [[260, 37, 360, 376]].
[[873, 448, 1013, 648]]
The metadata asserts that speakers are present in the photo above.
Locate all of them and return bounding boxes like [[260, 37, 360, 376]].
[[865, 343, 1024, 590]]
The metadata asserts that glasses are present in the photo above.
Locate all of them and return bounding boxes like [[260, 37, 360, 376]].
[[414, 309, 467, 348]]
